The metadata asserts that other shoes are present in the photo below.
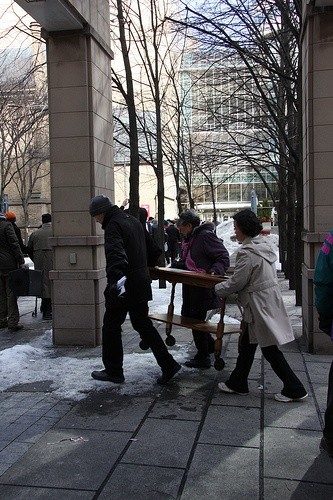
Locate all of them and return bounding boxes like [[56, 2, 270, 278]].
[[321, 436, 333, 457], [0, 320, 8, 328], [157, 366, 181, 384], [8, 324, 24, 331], [43, 313, 52, 320], [91, 370, 125, 383], [184, 358, 211, 369]]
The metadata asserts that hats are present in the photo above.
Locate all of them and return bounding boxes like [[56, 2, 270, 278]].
[[89, 194, 114, 217], [42, 213, 51, 224], [5, 212, 16, 222]]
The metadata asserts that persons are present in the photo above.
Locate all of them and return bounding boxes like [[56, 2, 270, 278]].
[[27, 213, 54, 319], [314, 232, 333, 449], [5, 211, 23, 252], [0, 212, 24, 330], [117, 198, 163, 263], [214, 210, 309, 402], [178, 210, 230, 368], [164, 218, 180, 264], [147, 216, 158, 241], [90, 196, 181, 384]]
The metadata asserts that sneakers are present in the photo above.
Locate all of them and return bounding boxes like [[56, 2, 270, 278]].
[[274, 393, 308, 402], [218, 382, 248, 396]]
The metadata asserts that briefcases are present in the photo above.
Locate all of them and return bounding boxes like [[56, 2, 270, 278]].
[[9, 264, 43, 296]]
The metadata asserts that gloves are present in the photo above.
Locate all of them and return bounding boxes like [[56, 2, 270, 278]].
[[112, 284, 122, 296]]
